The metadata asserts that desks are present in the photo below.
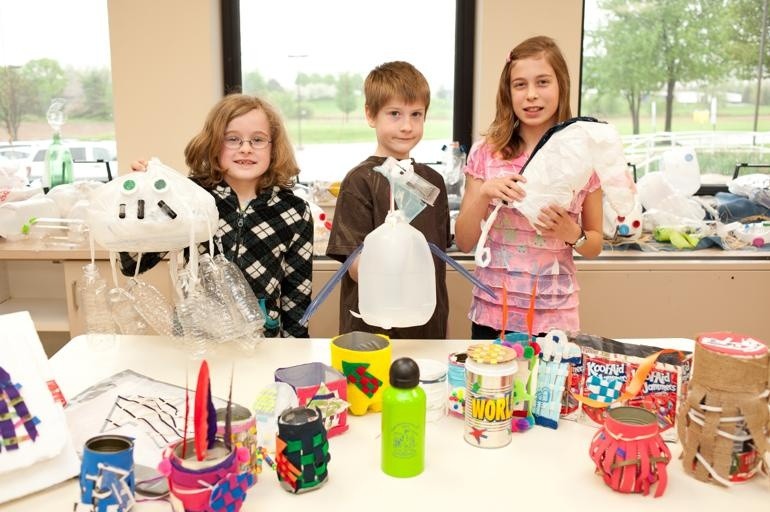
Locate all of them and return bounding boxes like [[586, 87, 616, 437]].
[[1, 333, 768, 510]]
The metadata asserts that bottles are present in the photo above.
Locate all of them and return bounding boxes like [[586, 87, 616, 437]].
[[81, 253, 270, 364], [379, 356, 426, 478], [46, 133, 75, 188]]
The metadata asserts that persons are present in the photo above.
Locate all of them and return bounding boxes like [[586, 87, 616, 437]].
[[451, 34, 607, 342], [111, 93, 315, 341], [323, 59, 453, 341]]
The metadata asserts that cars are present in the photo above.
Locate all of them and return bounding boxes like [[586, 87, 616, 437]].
[[0, 140, 117, 188]]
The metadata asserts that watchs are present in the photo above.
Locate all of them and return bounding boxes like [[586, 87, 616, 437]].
[[564, 228, 590, 250]]
